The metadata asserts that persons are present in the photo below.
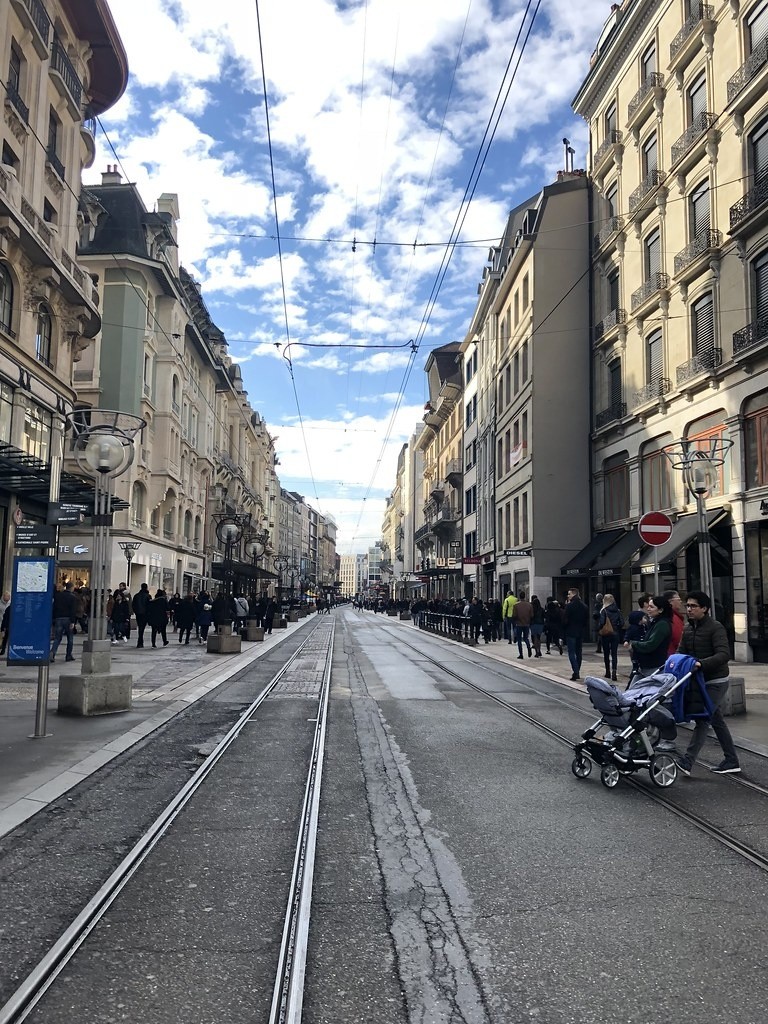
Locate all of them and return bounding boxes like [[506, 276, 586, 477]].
[[132, 583, 152, 648], [0, 590, 11, 655], [592, 593, 611, 654], [410, 590, 566, 661], [148, 589, 169, 649], [161, 582, 313, 646], [110, 593, 130, 644], [599, 594, 624, 681], [564, 587, 588, 680], [314, 595, 415, 618], [52, 579, 134, 656], [623, 589, 685, 752], [51, 582, 79, 662], [674, 591, 742, 776]]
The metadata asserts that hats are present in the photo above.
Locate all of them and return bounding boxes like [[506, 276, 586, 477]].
[[628, 611, 646, 625]]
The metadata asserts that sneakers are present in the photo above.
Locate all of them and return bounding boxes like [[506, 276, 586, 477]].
[[674, 759, 694, 776], [710, 758, 742, 774]]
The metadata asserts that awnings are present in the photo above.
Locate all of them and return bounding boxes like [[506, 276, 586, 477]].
[[560, 508, 729, 578]]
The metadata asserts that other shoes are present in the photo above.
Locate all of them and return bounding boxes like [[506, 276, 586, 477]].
[[498, 635, 502, 640], [65, 654, 76, 662], [508, 638, 512, 644], [534, 652, 538, 657], [185, 637, 190, 644], [513, 636, 517, 643], [517, 655, 523, 659], [137, 643, 144, 648], [268, 630, 272, 634], [492, 638, 496, 642], [539, 652, 542, 656], [199, 636, 203, 644], [151, 643, 157, 648], [122, 635, 129, 643], [570, 673, 581, 680], [49, 649, 57, 663], [111, 640, 121, 645], [475, 637, 479, 645], [163, 641, 170, 646], [179, 638, 183, 643], [545, 651, 551, 654], [528, 652, 532, 657], [0, 646, 6, 655], [558, 646, 564, 655]]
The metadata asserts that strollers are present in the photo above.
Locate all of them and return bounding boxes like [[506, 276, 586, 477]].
[[571, 654, 699, 790]]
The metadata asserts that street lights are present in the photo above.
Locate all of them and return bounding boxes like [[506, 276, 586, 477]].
[[286, 564, 301, 622], [661, 437, 749, 716], [118, 541, 142, 630], [299, 574, 318, 616], [243, 534, 271, 641], [273, 553, 291, 628], [374, 588, 383, 612], [208, 511, 250, 652], [399, 571, 413, 621], [388, 578, 398, 616], [57, 409, 147, 717]]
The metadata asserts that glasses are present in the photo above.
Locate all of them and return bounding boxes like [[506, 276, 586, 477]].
[[684, 604, 705, 609], [668, 597, 681, 602], [117, 595, 120, 598]]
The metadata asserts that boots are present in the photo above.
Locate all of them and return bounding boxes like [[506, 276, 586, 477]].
[[604, 660, 611, 678], [612, 669, 617, 681]]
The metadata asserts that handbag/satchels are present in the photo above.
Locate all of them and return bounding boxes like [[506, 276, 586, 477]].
[[598, 609, 613, 636]]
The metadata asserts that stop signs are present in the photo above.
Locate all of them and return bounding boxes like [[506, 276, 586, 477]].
[[638, 510, 674, 547]]
